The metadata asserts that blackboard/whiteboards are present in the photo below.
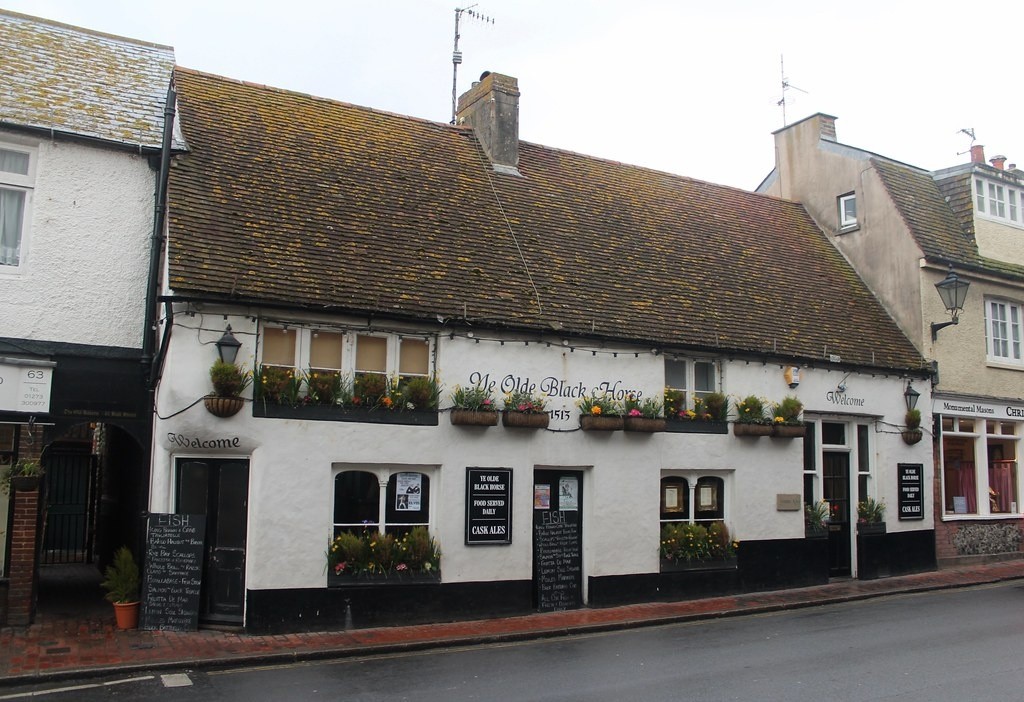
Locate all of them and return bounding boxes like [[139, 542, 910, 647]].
[[534, 510, 583, 614], [139, 513, 207, 632]]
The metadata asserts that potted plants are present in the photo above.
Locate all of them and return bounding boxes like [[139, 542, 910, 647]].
[[1, 459, 43, 495], [901, 410, 922, 446], [98, 548, 141, 628]]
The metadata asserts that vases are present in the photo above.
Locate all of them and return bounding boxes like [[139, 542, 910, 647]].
[[451, 407, 499, 426], [622, 414, 661, 432], [771, 422, 807, 438], [806, 526, 829, 537], [501, 409, 550, 428], [205, 397, 244, 417], [858, 522, 886, 534], [580, 414, 624, 431], [734, 421, 774, 437]]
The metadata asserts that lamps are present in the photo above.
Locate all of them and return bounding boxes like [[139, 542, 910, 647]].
[[906, 383, 920, 410], [214, 328, 240, 359], [838, 371, 851, 392], [931, 265, 970, 342]]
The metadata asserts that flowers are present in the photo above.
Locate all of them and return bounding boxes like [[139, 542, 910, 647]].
[[449, 383, 497, 412], [575, 392, 624, 418], [858, 501, 885, 523], [625, 391, 664, 417], [209, 357, 254, 396], [661, 521, 738, 565], [261, 365, 441, 415], [770, 397, 803, 421], [805, 500, 828, 533], [664, 387, 734, 420], [502, 391, 549, 415], [328, 527, 440, 578], [735, 395, 770, 420]]
